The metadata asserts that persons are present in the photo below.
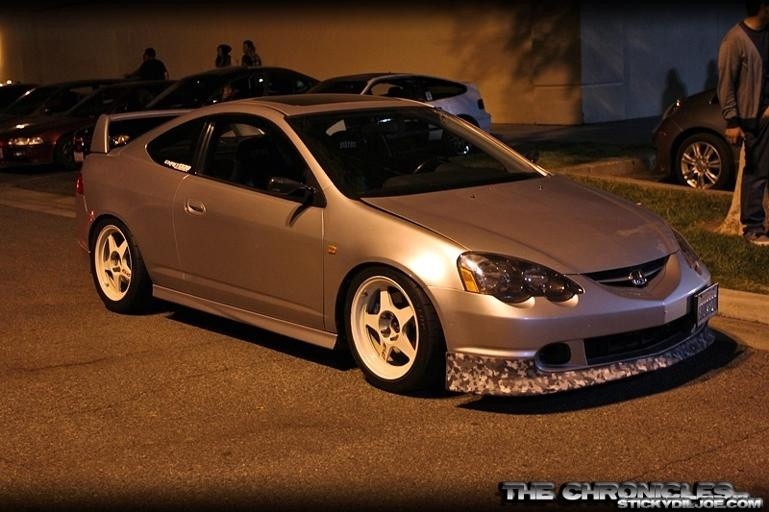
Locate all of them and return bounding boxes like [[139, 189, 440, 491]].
[[214, 43, 231, 69], [122, 46, 170, 81], [240, 41, 262, 67], [716, 1, 769, 247]]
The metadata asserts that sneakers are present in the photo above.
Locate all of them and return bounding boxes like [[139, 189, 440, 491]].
[[743, 225, 768, 247]]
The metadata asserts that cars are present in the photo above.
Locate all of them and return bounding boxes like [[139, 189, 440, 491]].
[[78, 93, 720, 397], [640, 83, 768, 191]]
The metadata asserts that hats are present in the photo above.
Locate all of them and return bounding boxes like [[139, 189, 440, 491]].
[[218, 45, 232, 56]]
